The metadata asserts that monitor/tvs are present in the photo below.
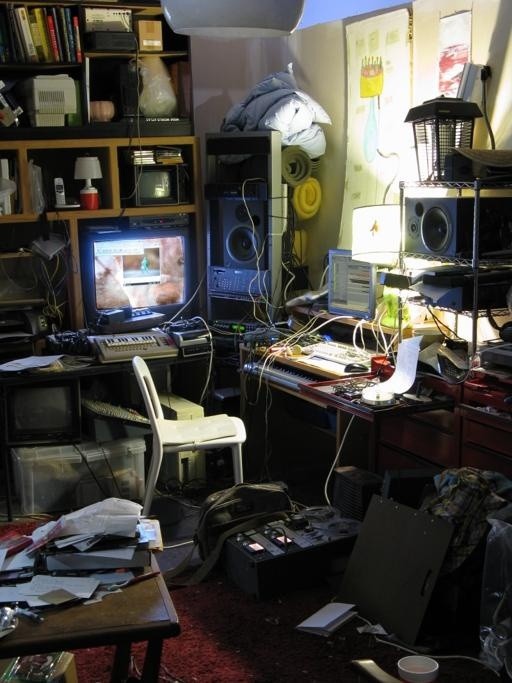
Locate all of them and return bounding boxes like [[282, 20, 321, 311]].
[[3, 377, 83, 445], [328, 249, 378, 320], [78, 227, 192, 329]]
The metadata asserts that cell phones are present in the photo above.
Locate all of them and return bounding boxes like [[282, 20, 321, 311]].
[[54, 178, 65, 205]]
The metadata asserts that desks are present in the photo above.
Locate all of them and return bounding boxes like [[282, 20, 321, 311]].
[[238, 306, 456, 468], [0, 336, 217, 521], [0, 547, 182, 683]]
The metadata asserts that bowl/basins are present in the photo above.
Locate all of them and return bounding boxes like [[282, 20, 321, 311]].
[[412, 323, 446, 350], [89, 99, 115, 122]]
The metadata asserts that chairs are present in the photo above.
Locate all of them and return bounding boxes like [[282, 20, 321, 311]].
[[131, 355, 247, 516]]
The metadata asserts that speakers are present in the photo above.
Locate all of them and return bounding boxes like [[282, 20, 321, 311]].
[[210, 195, 266, 270], [405, 196, 512, 259]]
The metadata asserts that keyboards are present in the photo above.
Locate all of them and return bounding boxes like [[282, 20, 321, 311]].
[[301, 340, 375, 366]]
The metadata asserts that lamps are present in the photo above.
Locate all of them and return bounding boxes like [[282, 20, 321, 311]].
[[74, 152, 103, 210], [404, 94, 483, 184], [160, 0, 305, 39]]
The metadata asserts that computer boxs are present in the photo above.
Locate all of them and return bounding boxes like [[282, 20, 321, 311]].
[[156, 389, 207, 491]]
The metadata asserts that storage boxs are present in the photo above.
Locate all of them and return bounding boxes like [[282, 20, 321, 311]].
[[10, 438, 149, 515]]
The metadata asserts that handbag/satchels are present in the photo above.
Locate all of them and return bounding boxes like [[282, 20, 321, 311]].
[[162, 481, 308, 587]]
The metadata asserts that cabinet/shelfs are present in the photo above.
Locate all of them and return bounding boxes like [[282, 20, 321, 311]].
[[0, 135, 208, 356], [368, 371, 462, 471], [455, 372, 512, 481], [0, 0, 195, 140], [201, 131, 313, 321], [398, 179, 512, 359]]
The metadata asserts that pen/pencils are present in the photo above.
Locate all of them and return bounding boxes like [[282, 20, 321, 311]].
[[20, 610, 40, 621], [127, 571, 160, 584]]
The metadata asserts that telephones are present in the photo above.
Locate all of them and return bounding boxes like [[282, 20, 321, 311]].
[[54, 178, 80, 209]]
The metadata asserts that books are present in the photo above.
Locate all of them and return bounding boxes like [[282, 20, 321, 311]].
[[132, 144, 184, 164], [0, 2, 82, 61]]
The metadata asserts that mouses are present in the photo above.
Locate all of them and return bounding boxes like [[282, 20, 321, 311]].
[[344, 363, 368, 371]]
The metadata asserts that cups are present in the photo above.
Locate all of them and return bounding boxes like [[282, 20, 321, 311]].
[[398, 655, 440, 683]]
